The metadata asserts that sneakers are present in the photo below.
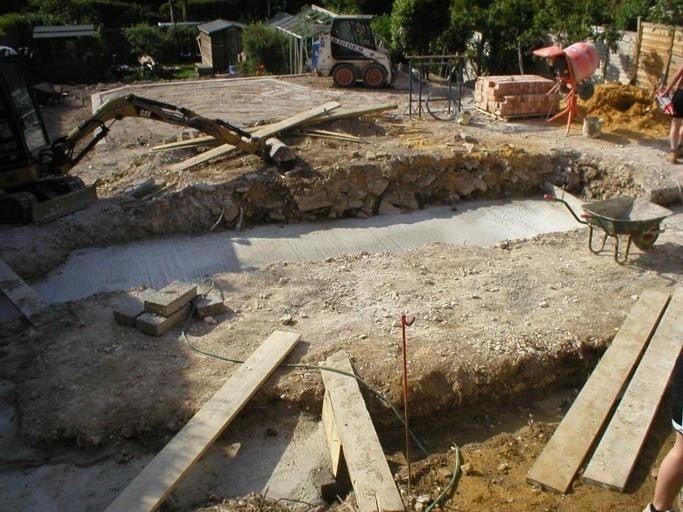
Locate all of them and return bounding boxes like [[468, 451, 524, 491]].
[[666, 146, 683, 165]]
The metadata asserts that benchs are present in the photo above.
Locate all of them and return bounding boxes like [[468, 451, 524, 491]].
[[196, 67, 217, 79]]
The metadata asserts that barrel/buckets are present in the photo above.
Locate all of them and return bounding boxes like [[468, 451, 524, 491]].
[[582, 115, 602, 137], [229, 64, 236, 77]]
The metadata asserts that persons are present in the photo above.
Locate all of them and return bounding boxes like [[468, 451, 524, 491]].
[[659, 65, 683, 165], [643, 357, 683, 512]]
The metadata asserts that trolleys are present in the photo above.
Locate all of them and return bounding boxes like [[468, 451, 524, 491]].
[[543, 180, 676, 269]]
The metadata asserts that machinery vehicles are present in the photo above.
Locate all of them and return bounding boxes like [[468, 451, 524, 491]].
[[1, 41, 308, 231], [307, 11, 436, 95]]
